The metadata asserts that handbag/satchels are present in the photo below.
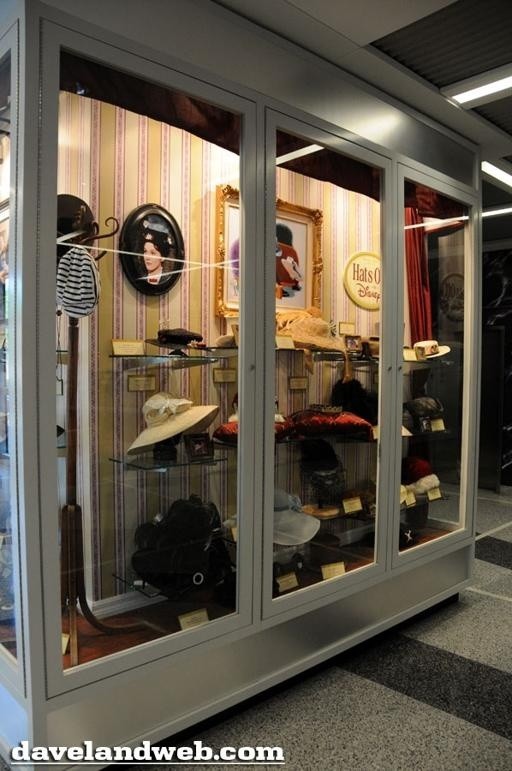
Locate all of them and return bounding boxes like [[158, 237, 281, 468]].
[[401, 395, 448, 434]]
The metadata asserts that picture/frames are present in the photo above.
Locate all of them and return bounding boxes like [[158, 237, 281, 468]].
[[213, 183, 323, 320], [118, 203, 185, 296]]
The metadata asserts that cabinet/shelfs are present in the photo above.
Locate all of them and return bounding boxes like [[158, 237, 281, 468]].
[[108, 345, 457, 634]]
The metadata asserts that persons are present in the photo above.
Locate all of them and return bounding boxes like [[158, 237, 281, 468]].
[[132, 230, 173, 284]]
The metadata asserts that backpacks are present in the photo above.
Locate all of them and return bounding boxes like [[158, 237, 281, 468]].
[[132, 496, 231, 601]]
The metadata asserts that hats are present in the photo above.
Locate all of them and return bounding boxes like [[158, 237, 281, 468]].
[[401, 455, 441, 495], [275, 316, 346, 353], [140, 227, 173, 259], [144, 328, 212, 353], [54, 246, 105, 320], [222, 488, 321, 547], [362, 521, 416, 547], [127, 389, 220, 456], [412, 339, 451, 359]]
